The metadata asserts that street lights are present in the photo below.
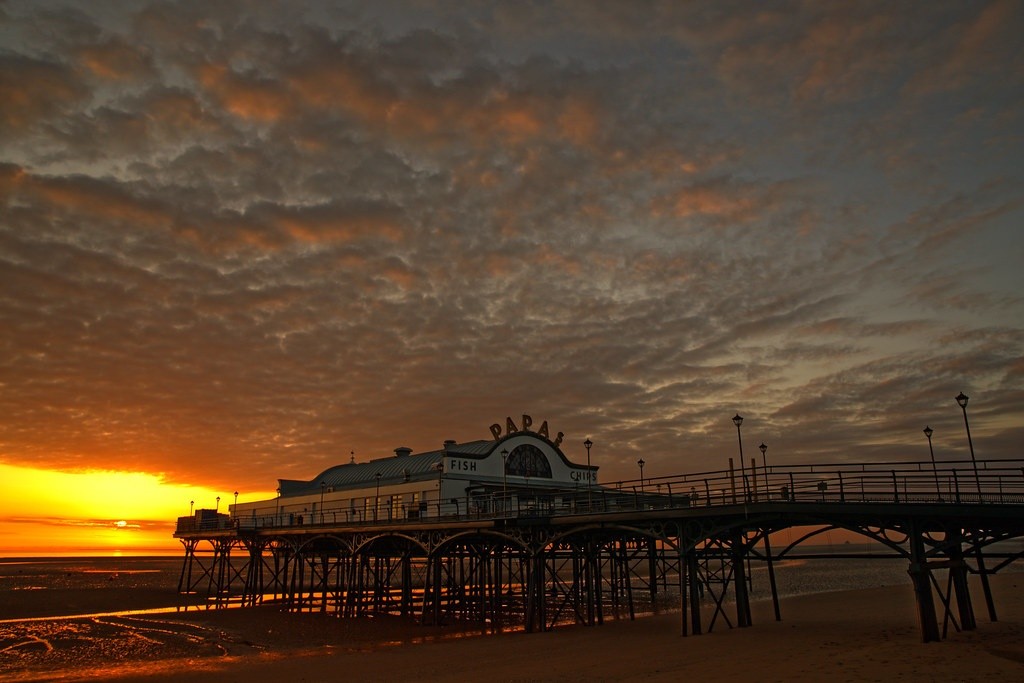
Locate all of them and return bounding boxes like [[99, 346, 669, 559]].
[[500, 448, 509, 517], [954, 391, 991, 504], [216, 496, 220, 510], [759, 442, 770, 500], [731, 412, 749, 502], [374, 472, 382, 523], [923, 425, 941, 500], [234, 491, 239, 517], [320, 481, 326, 524], [275, 487, 281, 528], [638, 458, 645, 496], [190, 500, 194, 514], [583, 437, 596, 511], [437, 462, 445, 522]]
